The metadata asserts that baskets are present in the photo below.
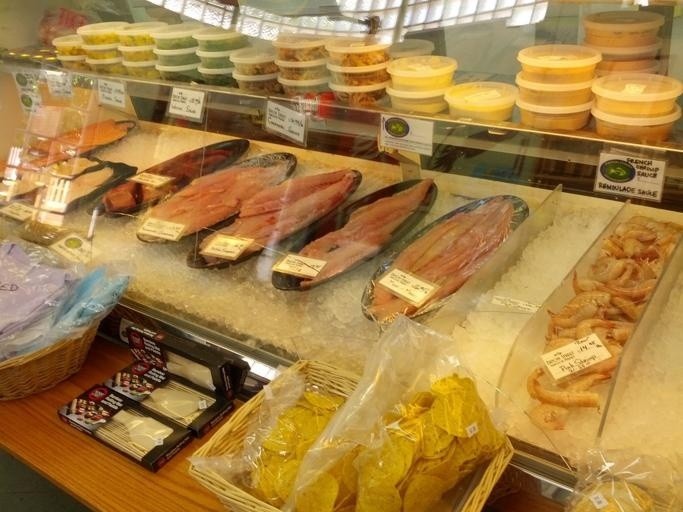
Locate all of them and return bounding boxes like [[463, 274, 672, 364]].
[[188, 357, 517, 512], [0, 323, 98, 402]]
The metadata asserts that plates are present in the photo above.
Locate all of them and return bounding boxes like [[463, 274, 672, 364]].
[[186, 169, 363, 272], [269, 176, 439, 294], [0, 121, 140, 222], [84, 140, 253, 221], [134, 152, 300, 246], [359, 195, 532, 327]]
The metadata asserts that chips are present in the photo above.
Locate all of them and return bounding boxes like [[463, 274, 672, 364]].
[[573, 483, 654, 512], [250, 383, 345, 507], [294, 375, 504, 512]]
